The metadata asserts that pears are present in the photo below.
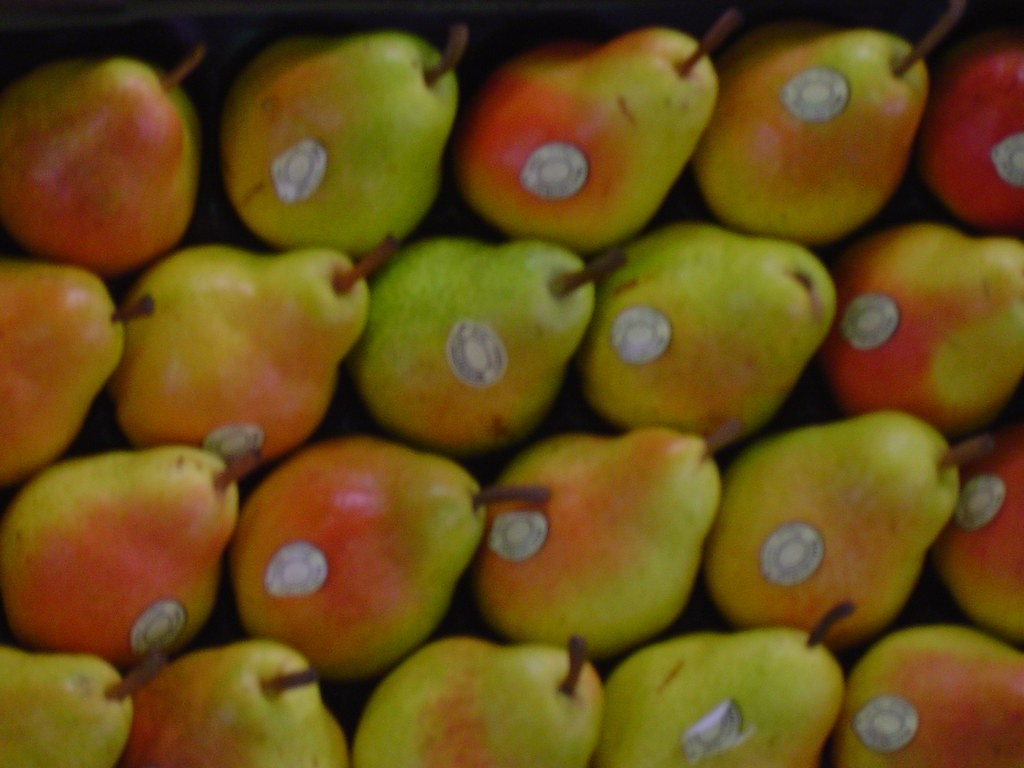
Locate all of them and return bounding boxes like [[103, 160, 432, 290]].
[[0, 0, 1022, 768]]
